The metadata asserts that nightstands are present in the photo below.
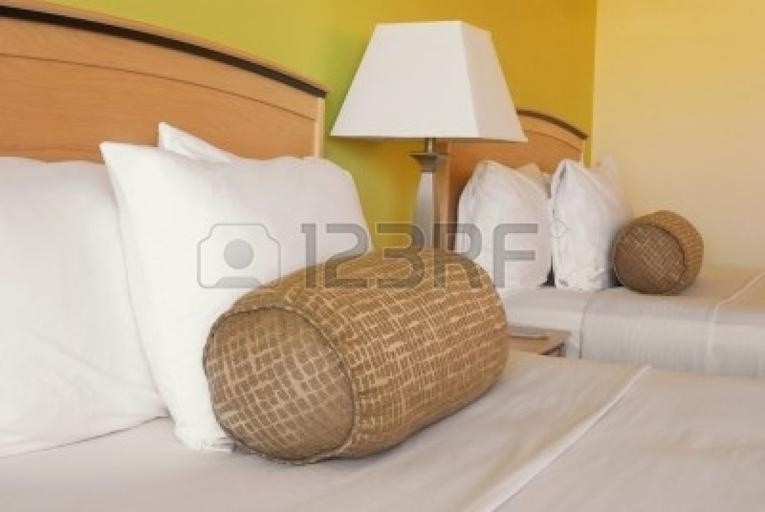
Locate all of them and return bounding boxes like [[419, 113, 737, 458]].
[[502, 323, 571, 355]]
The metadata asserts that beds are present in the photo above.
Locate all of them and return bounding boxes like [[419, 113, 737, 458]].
[[437, 109, 765, 376], [0, 0, 765, 511]]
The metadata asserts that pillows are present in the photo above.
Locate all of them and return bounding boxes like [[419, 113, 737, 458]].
[[454, 159, 703, 294]]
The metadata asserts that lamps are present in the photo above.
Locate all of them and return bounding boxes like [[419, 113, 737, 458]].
[[332, 22, 528, 246]]
